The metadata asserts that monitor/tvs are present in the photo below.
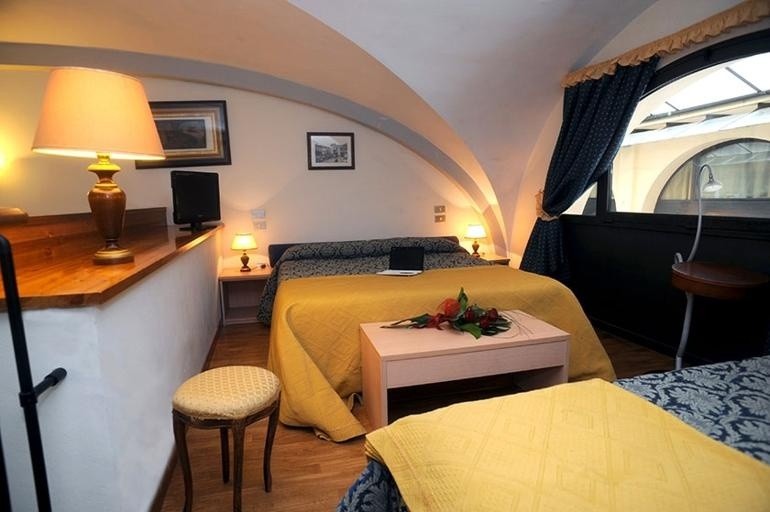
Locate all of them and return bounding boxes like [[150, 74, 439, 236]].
[[170, 169, 221, 231]]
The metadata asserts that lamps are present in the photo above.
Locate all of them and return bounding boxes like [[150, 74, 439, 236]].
[[231, 233, 258, 272], [464, 223, 487, 257], [674, 163, 722, 264], [31, 65, 166, 265]]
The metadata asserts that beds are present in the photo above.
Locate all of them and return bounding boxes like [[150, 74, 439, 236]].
[[256, 236, 618, 443], [334, 352, 770, 512]]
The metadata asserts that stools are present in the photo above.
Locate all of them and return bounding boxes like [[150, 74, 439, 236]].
[[171, 365, 283, 512]]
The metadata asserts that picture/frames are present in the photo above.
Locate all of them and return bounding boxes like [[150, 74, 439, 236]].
[[134, 99, 232, 170], [306, 131, 355, 170]]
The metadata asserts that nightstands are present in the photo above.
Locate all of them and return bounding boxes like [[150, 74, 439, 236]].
[[218, 263, 271, 328], [479, 253, 511, 266]]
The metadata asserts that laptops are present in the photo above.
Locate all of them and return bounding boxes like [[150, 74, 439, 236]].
[[376, 246, 424, 277]]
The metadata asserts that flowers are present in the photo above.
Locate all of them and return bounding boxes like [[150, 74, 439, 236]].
[[380, 287, 535, 340]]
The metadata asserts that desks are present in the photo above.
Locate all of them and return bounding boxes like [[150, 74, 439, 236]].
[[671, 261, 769, 370], [358, 308, 571, 431]]
